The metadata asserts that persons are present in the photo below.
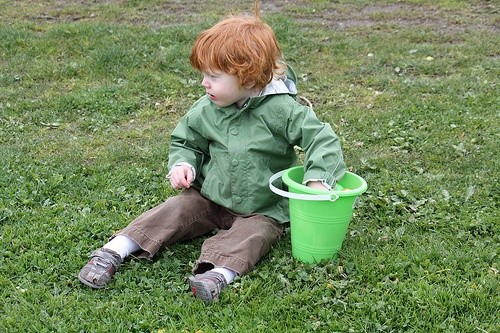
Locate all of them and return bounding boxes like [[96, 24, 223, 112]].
[[78, 15, 348, 305]]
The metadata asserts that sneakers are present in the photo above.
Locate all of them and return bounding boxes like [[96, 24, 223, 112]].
[[189, 271, 227, 306], [78, 247, 125, 288]]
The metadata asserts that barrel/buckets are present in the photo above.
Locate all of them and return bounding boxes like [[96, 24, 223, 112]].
[[268, 165, 367, 264]]
